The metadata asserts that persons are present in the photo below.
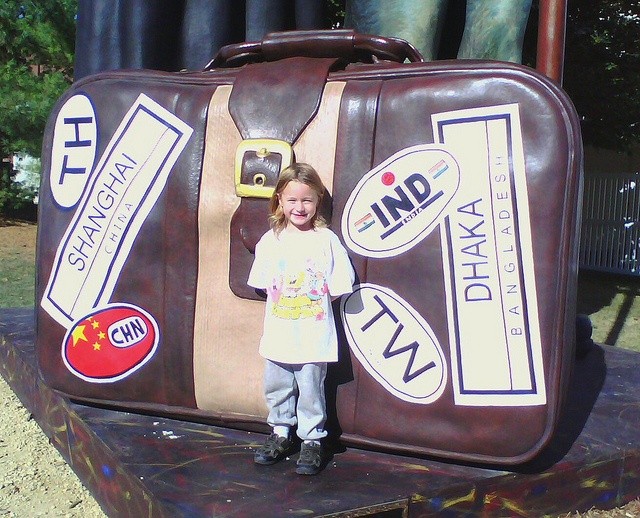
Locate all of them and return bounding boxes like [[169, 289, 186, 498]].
[[246, 163, 356, 474]]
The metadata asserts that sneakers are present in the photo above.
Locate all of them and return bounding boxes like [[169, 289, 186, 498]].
[[295, 441, 324, 475], [253, 433, 301, 466]]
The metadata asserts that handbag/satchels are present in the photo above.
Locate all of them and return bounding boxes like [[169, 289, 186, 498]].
[[33, 27, 586, 466]]
